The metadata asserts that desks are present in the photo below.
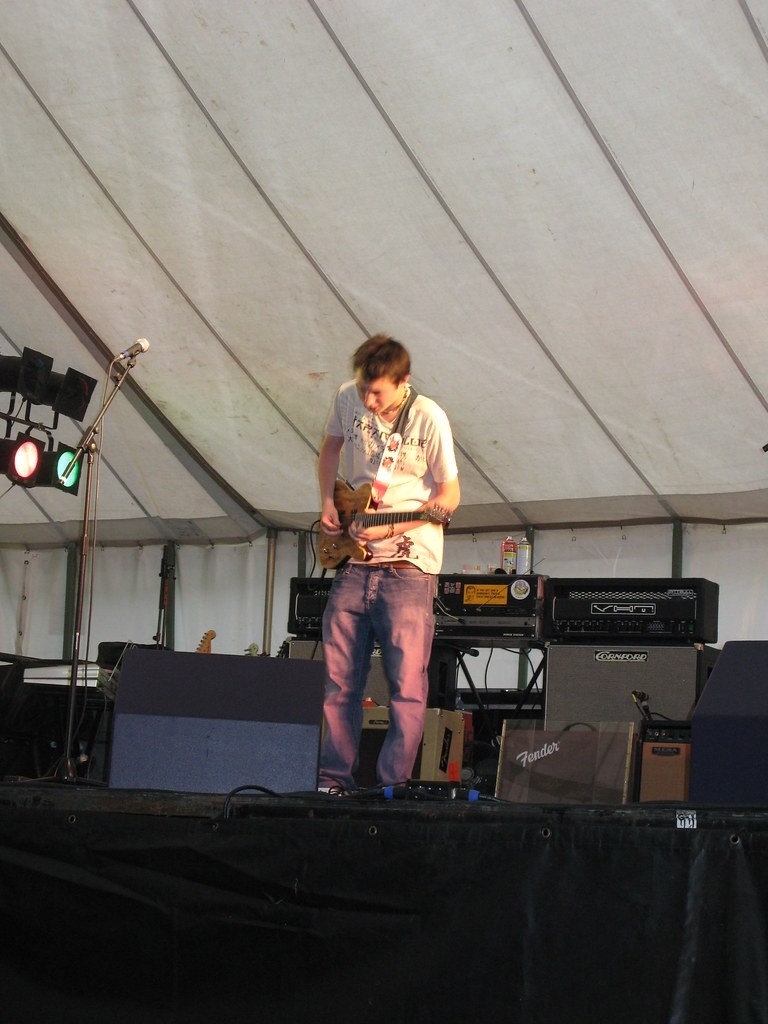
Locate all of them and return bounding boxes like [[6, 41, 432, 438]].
[[415, 636, 548, 786]]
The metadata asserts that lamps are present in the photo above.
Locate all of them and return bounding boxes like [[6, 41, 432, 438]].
[[0, 347, 53, 405], [38, 367, 98, 422], [0, 431, 46, 488], [37, 441, 84, 497]]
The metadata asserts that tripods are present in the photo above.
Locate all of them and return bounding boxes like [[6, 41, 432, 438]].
[[18, 346, 141, 786]]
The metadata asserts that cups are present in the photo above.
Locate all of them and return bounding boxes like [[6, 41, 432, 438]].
[[487, 563, 497, 574]]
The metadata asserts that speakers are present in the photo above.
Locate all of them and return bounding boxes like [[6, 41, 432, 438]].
[[494, 644, 768, 805], [108, 637, 467, 795]]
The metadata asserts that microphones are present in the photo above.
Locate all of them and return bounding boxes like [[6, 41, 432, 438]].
[[632, 690, 649, 701], [113, 338, 150, 362]]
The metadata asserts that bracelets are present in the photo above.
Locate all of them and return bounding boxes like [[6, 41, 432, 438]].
[[385, 523, 394, 539]]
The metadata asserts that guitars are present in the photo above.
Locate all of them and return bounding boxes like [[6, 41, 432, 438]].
[[195, 629, 217, 653], [277, 640, 287, 657], [244, 643, 259, 656], [317, 479, 454, 570]]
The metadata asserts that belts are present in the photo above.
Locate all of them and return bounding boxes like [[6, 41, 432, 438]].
[[363, 560, 419, 569]]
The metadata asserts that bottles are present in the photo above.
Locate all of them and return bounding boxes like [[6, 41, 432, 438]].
[[516, 537, 532, 575], [501, 536, 516, 573]]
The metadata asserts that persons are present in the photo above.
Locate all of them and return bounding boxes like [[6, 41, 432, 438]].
[[317, 333, 461, 794]]
